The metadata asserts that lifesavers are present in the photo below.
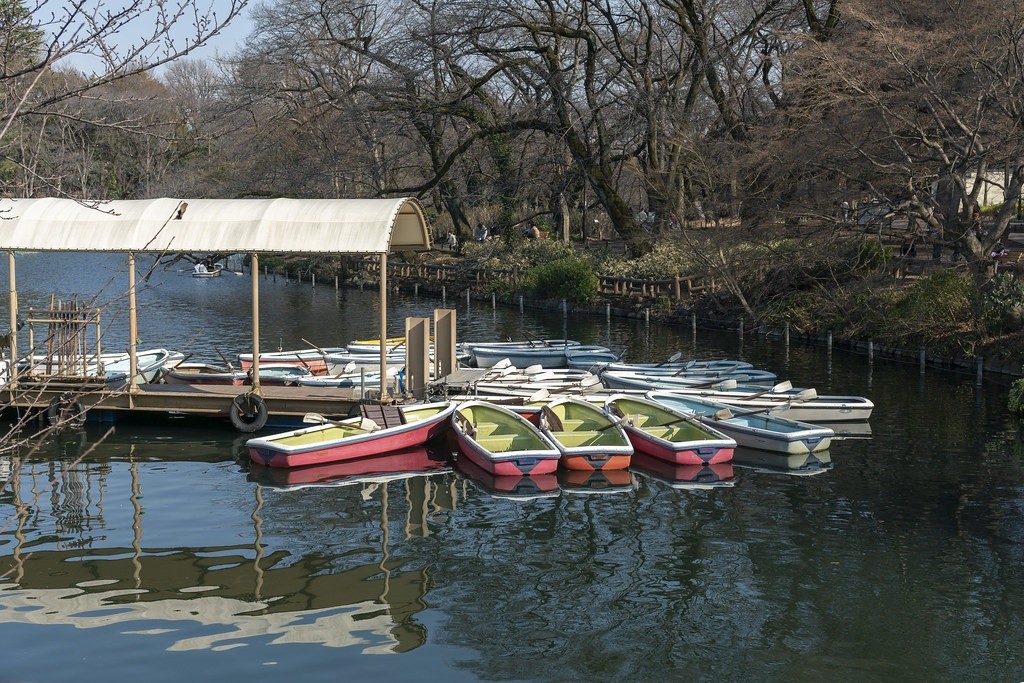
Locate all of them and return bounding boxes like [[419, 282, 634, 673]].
[[229, 393, 270, 433], [47, 395, 86, 428], [232, 435, 258, 468], [54, 429, 87, 453]]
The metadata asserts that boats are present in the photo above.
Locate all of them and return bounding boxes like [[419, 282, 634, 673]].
[[449, 401, 561, 476], [557, 464, 638, 496], [541, 396, 634, 470], [733, 442, 836, 480], [631, 451, 740, 495], [642, 390, 836, 454], [603, 392, 738, 466], [1, 195, 458, 428], [191, 263, 224, 279], [439, 437, 563, 504], [809, 419, 875, 444], [244, 400, 458, 476], [704, 386, 876, 423], [21, 330, 782, 407], [239, 437, 451, 492]]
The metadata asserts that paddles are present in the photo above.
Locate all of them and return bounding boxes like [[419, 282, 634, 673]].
[[655, 352, 682, 368], [597, 413, 630, 431], [658, 410, 706, 426], [509, 363, 543, 375], [491, 357, 512, 370], [516, 370, 555, 384], [523, 387, 551, 405], [699, 379, 738, 389], [550, 374, 600, 394], [301, 412, 369, 431], [334, 360, 357, 379], [360, 416, 385, 431], [672, 359, 697, 377], [705, 403, 791, 418], [486, 365, 517, 383], [742, 380, 793, 401], [774, 387, 818, 402]]
